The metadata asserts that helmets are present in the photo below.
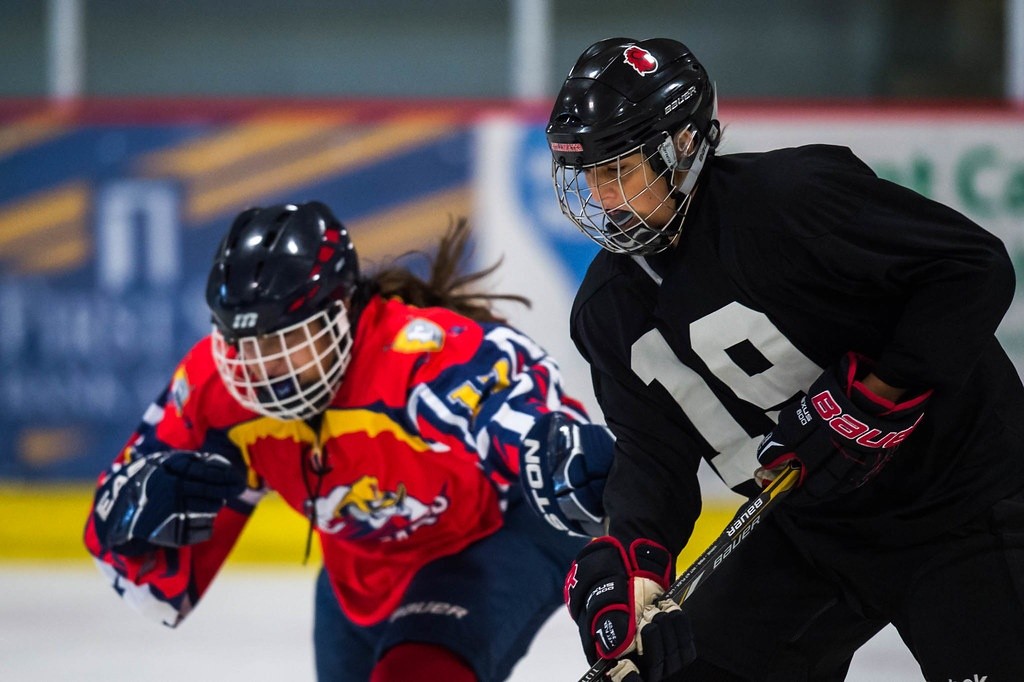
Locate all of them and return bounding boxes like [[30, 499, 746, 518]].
[[205, 201, 362, 422], [544, 37, 722, 253]]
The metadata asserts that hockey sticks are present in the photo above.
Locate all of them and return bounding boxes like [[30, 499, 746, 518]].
[[576, 463, 803, 682]]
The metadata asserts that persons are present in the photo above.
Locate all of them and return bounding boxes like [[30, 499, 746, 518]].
[[82, 201, 619, 682], [547, 37, 1024, 682]]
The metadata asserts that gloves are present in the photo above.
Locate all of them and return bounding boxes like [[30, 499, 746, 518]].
[[561, 538, 698, 682], [107, 451, 235, 555], [541, 414, 619, 537], [751, 352, 932, 507]]
